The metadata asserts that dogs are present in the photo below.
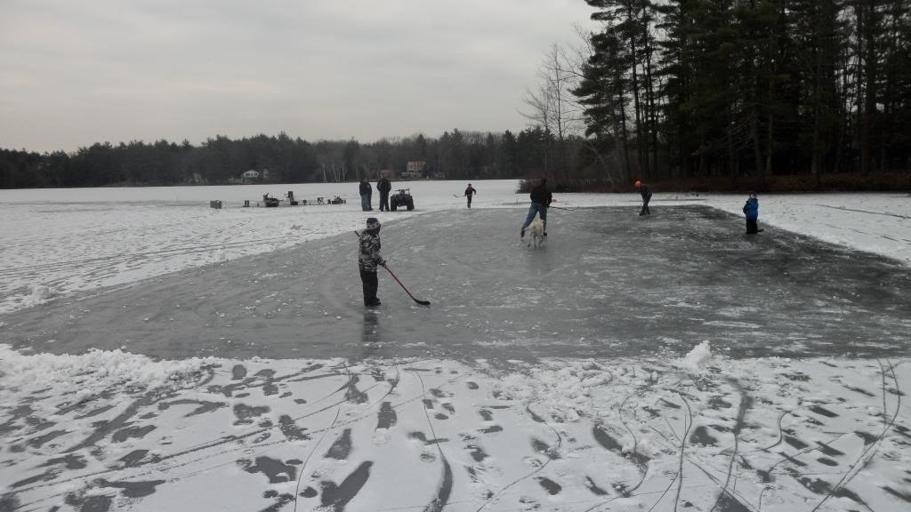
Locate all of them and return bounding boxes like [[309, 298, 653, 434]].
[[522, 218, 546, 248]]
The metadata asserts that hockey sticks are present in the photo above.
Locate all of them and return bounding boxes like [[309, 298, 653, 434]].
[[353, 229, 430, 306]]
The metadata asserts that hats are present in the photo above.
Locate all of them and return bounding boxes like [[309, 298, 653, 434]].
[[366, 218, 381, 230]]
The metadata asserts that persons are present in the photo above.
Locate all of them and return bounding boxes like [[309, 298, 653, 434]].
[[376, 169, 392, 212], [633, 179, 652, 216], [357, 218, 387, 307], [521, 179, 552, 237], [743, 192, 759, 235], [464, 184, 476, 208], [359, 175, 373, 211]]
